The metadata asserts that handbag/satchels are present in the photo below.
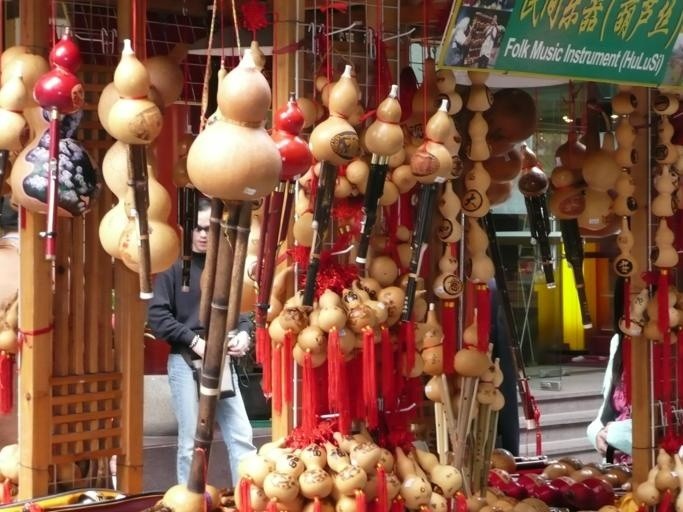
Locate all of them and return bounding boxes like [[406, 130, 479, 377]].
[[193, 360, 236, 400]]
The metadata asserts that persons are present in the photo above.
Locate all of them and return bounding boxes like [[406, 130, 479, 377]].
[[147, 199, 257, 495], [585, 276, 635, 469], [477, 13, 500, 69], [444, 7, 477, 66]]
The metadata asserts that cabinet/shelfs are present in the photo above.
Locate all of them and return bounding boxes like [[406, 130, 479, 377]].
[[497, 230, 564, 366]]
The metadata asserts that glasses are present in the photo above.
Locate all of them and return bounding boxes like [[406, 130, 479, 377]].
[[194, 224, 209, 233]]
